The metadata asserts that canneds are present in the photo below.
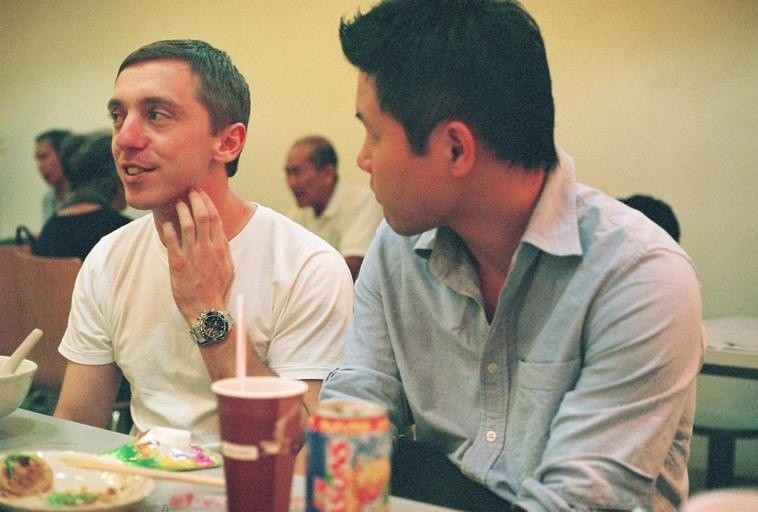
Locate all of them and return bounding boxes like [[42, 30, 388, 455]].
[[304, 398, 391, 511]]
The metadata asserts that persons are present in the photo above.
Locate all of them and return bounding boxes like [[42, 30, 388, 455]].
[[52, 36, 355, 451], [37, 129, 134, 257], [323, 1, 706, 509], [31, 125, 74, 224], [280, 132, 383, 258]]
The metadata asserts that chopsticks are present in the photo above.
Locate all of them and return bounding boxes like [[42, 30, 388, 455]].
[[64, 456, 230, 486]]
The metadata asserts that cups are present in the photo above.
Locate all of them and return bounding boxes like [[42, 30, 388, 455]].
[[211, 377, 310, 512]]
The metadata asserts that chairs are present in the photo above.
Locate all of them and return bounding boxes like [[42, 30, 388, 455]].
[[8, 243, 85, 392]]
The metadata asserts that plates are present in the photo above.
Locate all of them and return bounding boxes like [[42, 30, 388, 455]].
[[0, 450, 154, 511]]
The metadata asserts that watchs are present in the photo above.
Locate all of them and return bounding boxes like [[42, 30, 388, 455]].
[[184, 310, 236, 346]]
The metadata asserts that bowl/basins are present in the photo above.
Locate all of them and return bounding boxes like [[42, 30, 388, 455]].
[[0, 356, 37, 420]]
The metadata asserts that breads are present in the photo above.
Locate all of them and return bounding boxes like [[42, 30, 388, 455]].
[[0, 451, 52, 498]]
